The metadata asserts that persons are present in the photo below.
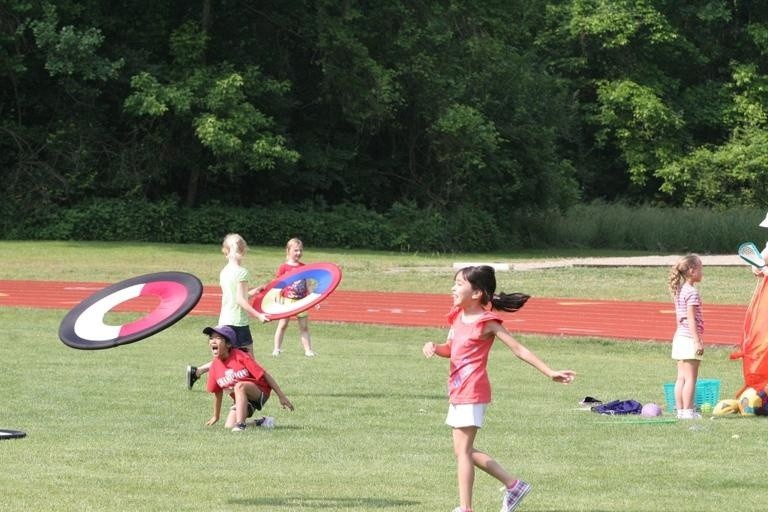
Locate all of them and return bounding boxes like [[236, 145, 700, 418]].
[[201, 323, 296, 433], [274, 237, 321, 358], [667, 253, 706, 418], [422, 266, 576, 511], [183, 232, 270, 391], [750, 212, 768, 277]]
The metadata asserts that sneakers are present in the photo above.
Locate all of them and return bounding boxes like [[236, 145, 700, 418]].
[[678, 414, 701, 419], [500, 480, 531, 512], [187, 366, 197, 390], [260, 416, 274, 428], [272, 350, 280, 356], [232, 423, 246, 432], [305, 351, 313, 357], [452, 507, 472, 512]]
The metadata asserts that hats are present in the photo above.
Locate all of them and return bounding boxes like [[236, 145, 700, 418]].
[[203, 325, 237, 345]]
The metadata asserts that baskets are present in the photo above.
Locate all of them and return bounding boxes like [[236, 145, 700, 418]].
[[665, 379, 721, 412]]
